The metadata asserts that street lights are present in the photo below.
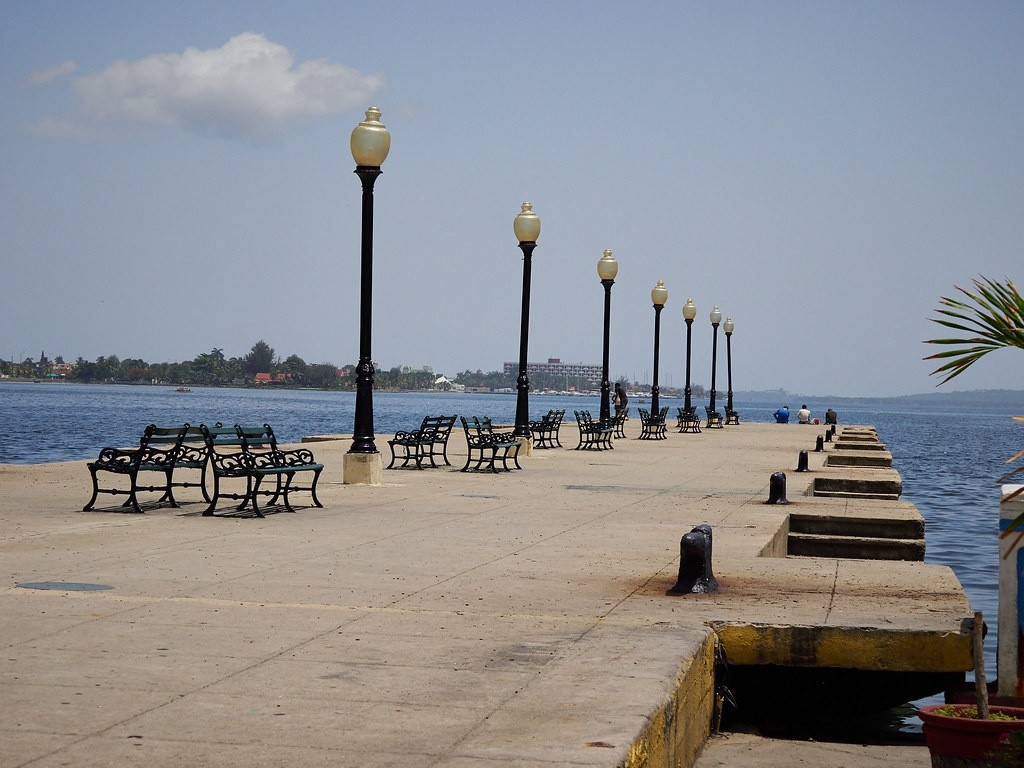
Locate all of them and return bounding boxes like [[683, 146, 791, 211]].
[[595, 249, 619, 426], [346, 105, 394, 454], [723, 317, 736, 412], [709, 305, 722, 412], [682, 298, 697, 415], [510, 201, 542, 436], [648, 279, 668, 419]]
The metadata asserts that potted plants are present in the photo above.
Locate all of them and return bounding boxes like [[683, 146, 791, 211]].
[[918, 609, 1024, 768]]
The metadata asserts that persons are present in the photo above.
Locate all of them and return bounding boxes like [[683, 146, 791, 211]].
[[774, 405, 790, 423], [797, 405, 811, 424], [612, 383, 628, 416], [825, 408, 837, 425]]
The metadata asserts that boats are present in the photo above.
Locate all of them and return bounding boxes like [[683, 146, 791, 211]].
[[176, 386, 194, 393]]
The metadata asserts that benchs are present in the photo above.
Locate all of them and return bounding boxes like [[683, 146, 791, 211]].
[[529, 409, 566, 450], [460, 416, 522, 474], [574, 406, 740, 451], [386, 413, 457, 469], [83, 422, 223, 514], [200, 423, 324, 517]]
